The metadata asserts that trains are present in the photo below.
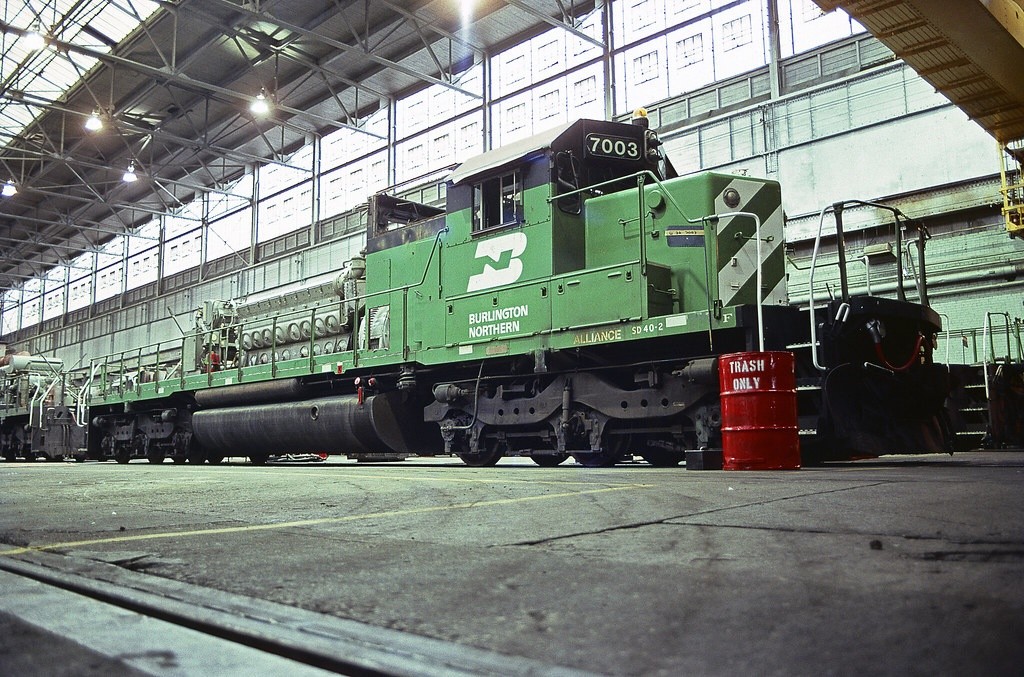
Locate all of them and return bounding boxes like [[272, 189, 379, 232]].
[[1, 108, 959, 472]]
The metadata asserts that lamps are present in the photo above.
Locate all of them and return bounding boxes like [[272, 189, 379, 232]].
[[121, 163, 139, 184], [85, 109, 103, 131], [252, 84, 271, 118], [2, 179, 19, 198]]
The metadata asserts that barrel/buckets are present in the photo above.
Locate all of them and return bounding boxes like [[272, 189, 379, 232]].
[[989, 364, 1024, 445], [718, 350, 801, 470]]
